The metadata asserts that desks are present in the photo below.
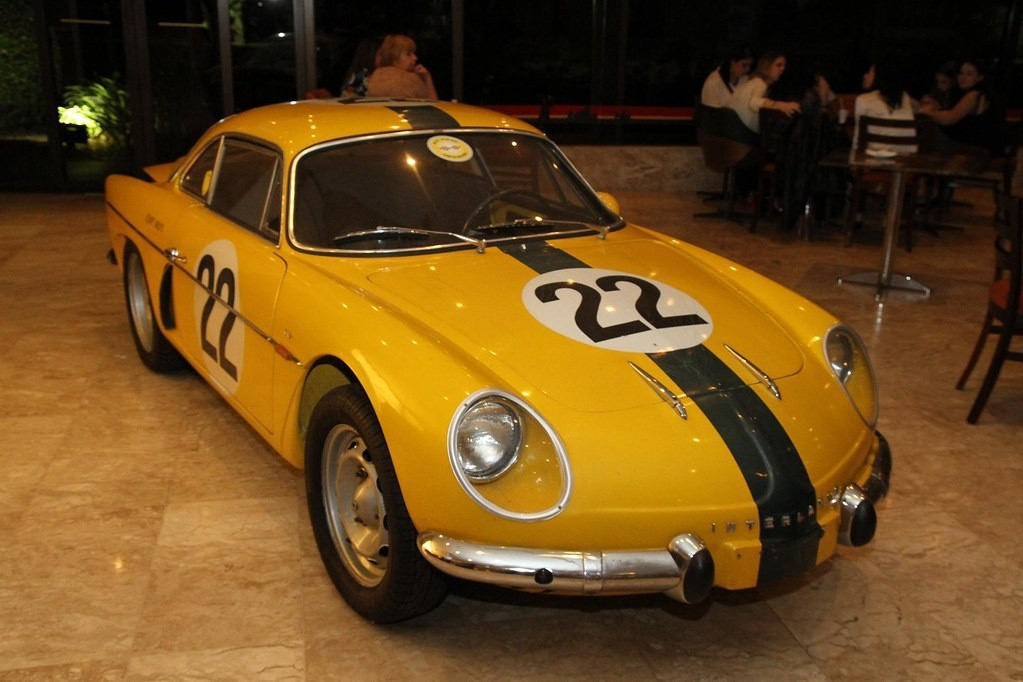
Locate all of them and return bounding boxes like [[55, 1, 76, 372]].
[[817, 145, 972, 306]]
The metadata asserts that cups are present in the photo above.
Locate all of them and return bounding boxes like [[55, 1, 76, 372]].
[[838, 109, 848, 124]]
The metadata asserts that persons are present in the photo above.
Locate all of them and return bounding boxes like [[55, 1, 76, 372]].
[[702, 43, 754, 108], [915, 56, 1002, 155], [848, 63, 918, 228], [805, 73, 843, 121], [920, 67, 958, 110], [727, 52, 803, 214], [342, 42, 384, 98], [364, 34, 438, 100], [352, 39, 376, 64]]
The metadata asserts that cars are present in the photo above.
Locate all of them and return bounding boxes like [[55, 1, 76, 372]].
[[103, 97, 895, 627]]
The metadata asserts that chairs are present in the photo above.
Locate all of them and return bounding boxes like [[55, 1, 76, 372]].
[[921, 103, 1021, 239], [832, 116, 928, 250], [955, 177, 1023, 428], [689, 103, 782, 228]]
